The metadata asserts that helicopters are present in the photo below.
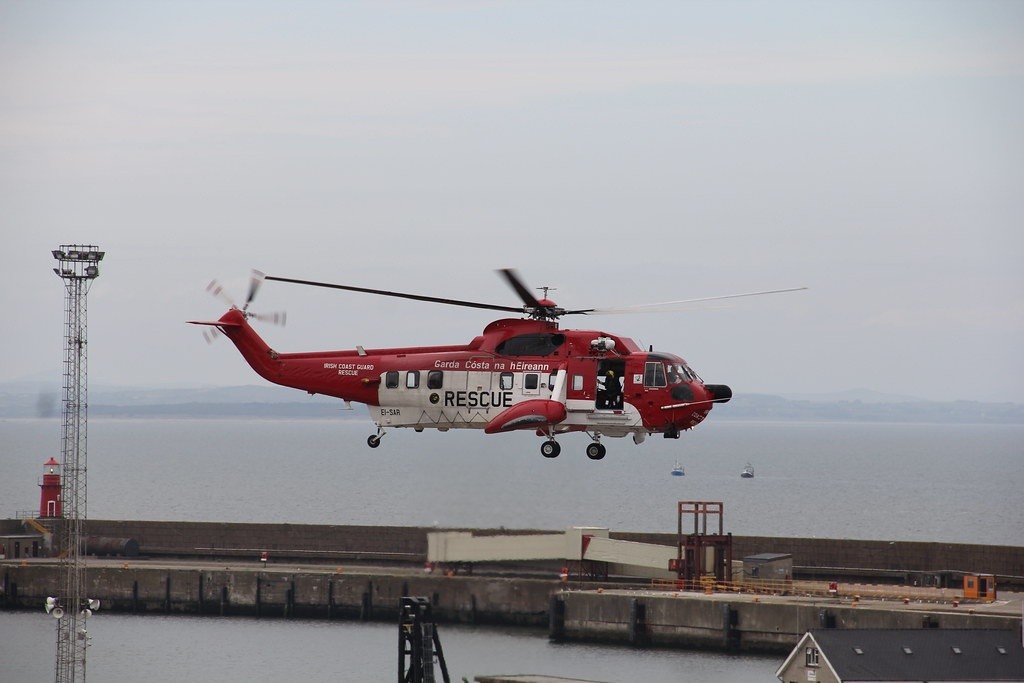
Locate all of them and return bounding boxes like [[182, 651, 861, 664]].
[[182, 258, 809, 462]]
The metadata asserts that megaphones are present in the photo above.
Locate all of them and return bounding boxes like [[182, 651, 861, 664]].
[[45, 597, 64, 618], [81, 598, 100, 618]]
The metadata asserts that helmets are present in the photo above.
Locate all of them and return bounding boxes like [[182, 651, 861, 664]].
[[605, 370, 614, 376]]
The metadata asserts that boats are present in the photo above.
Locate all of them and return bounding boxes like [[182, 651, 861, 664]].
[[669, 461, 686, 478], [740, 462, 755, 479]]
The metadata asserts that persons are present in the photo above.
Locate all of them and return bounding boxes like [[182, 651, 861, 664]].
[[596, 371, 621, 409], [668, 367, 682, 382]]
